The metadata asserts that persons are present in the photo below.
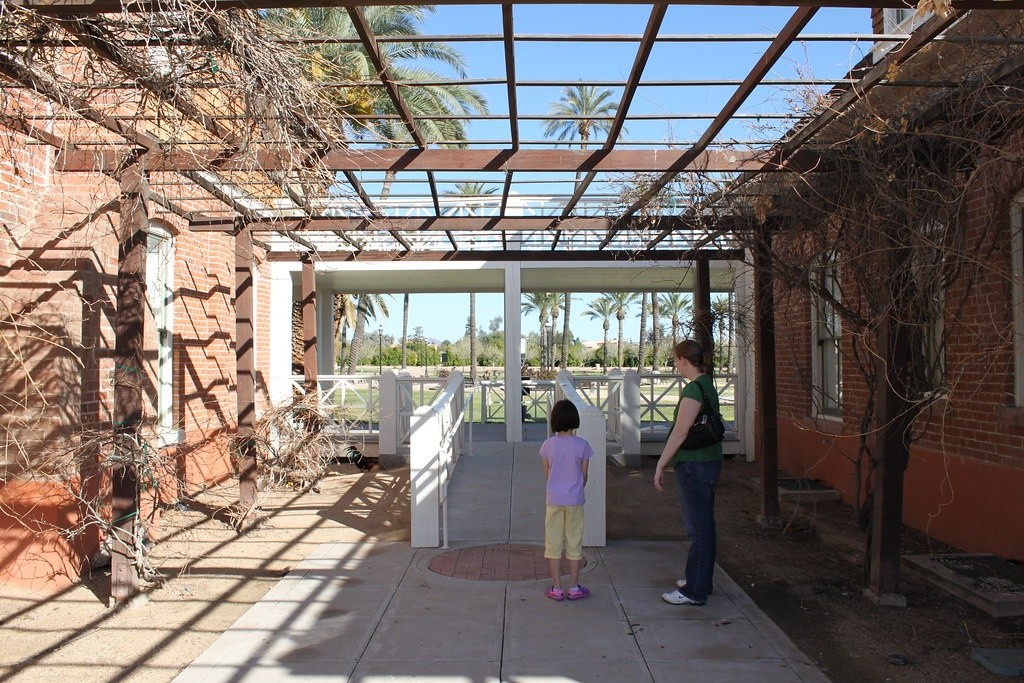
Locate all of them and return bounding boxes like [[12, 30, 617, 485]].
[[653, 338, 724, 604], [538, 398, 592, 601]]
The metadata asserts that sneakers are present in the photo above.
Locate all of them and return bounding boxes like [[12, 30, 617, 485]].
[[567, 584, 590, 601], [543, 585, 564, 602], [675, 578, 689, 588], [660, 589, 706, 606]]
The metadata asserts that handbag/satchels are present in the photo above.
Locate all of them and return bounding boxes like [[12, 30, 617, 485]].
[[667, 380, 725, 452]]
